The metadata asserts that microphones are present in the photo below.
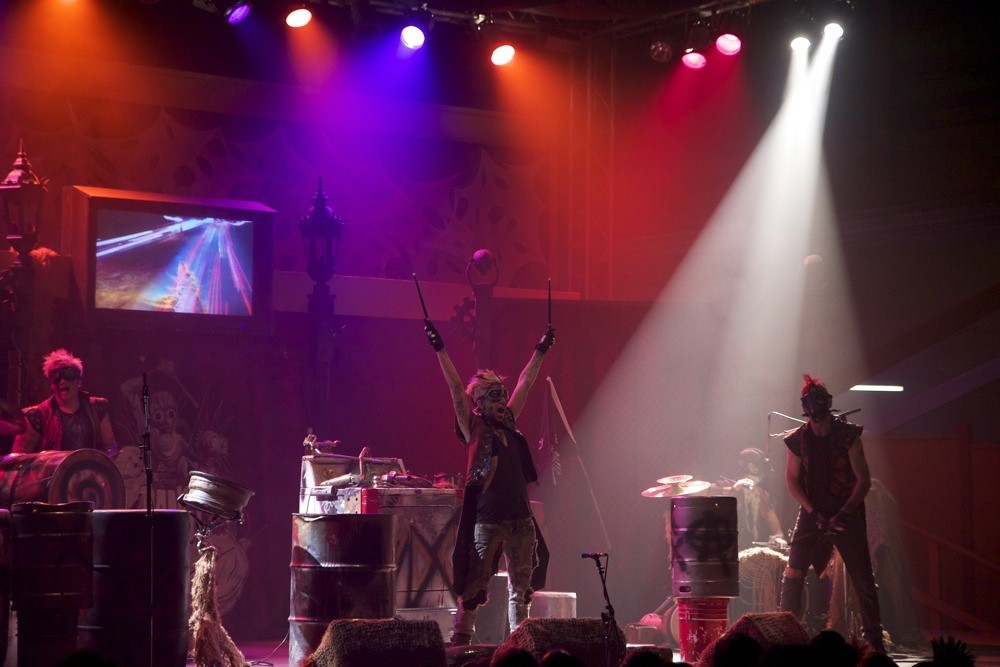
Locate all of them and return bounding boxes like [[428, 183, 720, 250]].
[[581, 551, 608, 559]]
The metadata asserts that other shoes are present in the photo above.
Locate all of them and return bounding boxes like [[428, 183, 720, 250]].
[[450, 633, 470, 647]]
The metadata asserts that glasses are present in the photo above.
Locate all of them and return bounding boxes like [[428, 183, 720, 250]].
[[49, 366, 82, 383], [478, 389, 507, 401]]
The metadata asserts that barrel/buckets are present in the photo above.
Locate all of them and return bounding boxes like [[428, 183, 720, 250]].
[[76, 509, 187, 667], [670, 496, 741, 598], [15, 608, 76, 666], [10, 502, 95, 608], [288, 513, 397, 666], [0, 449, 124, 508], [678, 598, 729, 662]]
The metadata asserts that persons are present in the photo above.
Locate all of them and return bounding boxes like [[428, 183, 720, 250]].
[[714, 630, 976, 667], [450, 640, 666, 667], [12, 347, 117, 453], [424, 325, 551, 646], [779, 376, 888, 655]]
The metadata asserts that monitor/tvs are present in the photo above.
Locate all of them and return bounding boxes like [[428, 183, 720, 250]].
[[59, 183, 278, 339]]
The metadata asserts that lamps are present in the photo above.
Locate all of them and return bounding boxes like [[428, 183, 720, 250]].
[[401, 6, 434, 50], [679, 9, 718, 69], [476, 20, 518, 67], [717, 2, 751, 57], [193, 0, 252, 25], [0, 136, 48, 263], [648, 24, 676, 62], [298, 175, 344, 295]]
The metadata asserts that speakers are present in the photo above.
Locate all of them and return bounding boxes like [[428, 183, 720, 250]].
[[489, 617, 627, 667], [701, 611, 811, 665], [303, 616, 446, 667]]
